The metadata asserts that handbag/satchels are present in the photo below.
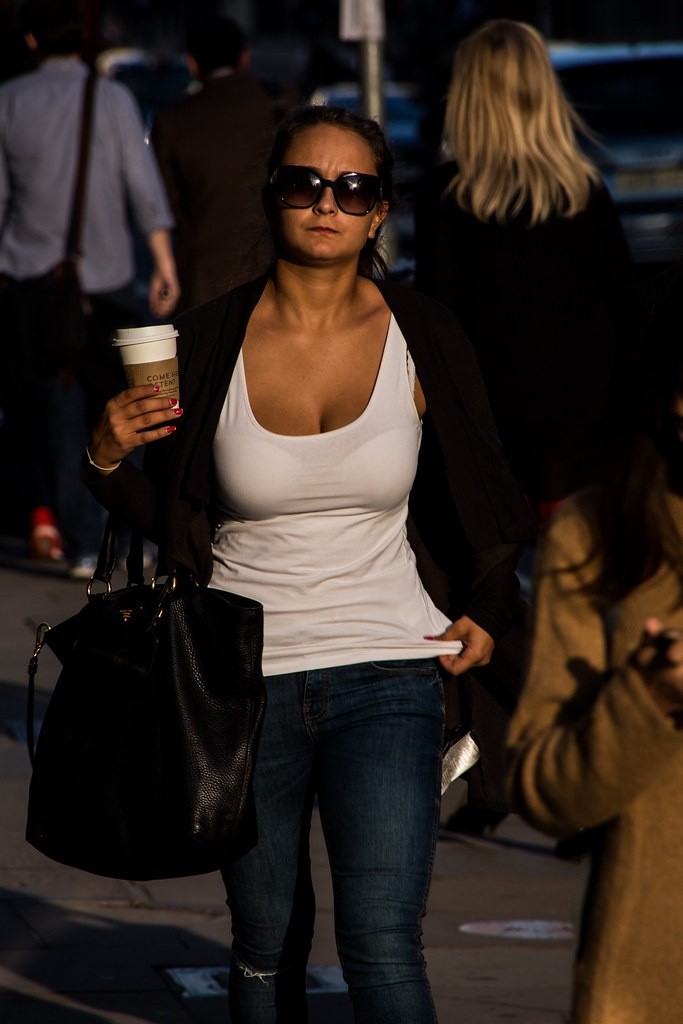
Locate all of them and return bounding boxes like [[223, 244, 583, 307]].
[[0, 259, 83, 381], [26, 467, 267, 882]]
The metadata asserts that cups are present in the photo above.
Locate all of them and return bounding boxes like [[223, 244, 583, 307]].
[[113, 324, 181, 411]]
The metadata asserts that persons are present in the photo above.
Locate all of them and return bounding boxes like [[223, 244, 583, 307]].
[[505, 360, 683, 1024], [48, 106, 521, 1024], [396, 19, 640, 866], [0, 0, 295, 579]]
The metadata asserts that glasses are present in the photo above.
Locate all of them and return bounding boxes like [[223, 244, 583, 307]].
[[269, 165, 383, 216]]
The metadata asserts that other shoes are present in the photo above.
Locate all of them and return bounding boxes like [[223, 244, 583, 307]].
[[116, 543, 153, 570], [445, 798, 514, 833], [28, 522, 64, 559], [67, 554, 97, 579]]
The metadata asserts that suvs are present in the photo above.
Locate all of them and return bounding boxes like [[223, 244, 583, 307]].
[[311, 40, 683, 265]]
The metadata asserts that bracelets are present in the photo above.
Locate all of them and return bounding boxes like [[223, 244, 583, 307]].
[[85, 435, 121, 471]]
